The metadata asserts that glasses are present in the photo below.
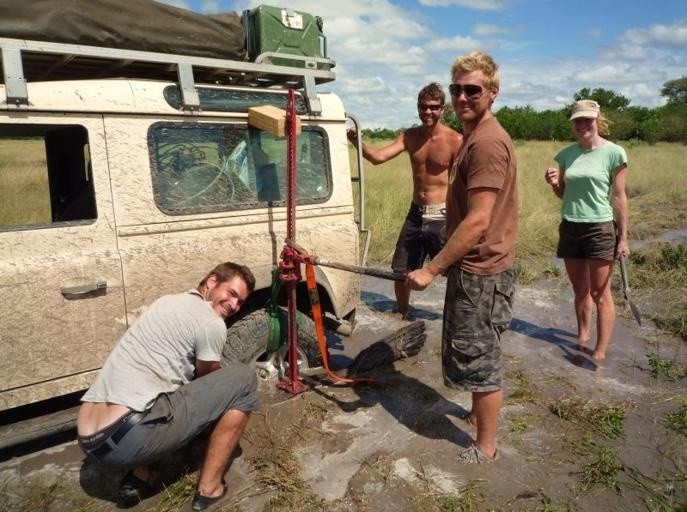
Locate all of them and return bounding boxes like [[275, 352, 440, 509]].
[[418, 104, 442, 110], [448, 84, 491, 97]]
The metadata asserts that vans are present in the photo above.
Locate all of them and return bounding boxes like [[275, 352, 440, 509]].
[[0, 32, 371, 453]]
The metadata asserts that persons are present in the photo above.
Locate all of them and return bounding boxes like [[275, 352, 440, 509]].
[[544, 99, 631, 362], [74, 261, 263, 511], [348, 80, 464, 316], [401, 50, 521, 467]]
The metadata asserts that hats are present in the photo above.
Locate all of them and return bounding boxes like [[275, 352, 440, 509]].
[[568, 100, 600, 120]]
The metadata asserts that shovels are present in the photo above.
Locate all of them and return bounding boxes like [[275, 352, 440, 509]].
[[614, 223, 645, 326]]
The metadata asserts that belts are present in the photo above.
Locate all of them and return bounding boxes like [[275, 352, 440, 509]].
[[81, 412, 145, 462]]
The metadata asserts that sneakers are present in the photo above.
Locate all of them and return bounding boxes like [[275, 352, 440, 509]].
[[455, 443, 501, 464], [192, 482, 228, 512], [116, 473, 151, 508]]
